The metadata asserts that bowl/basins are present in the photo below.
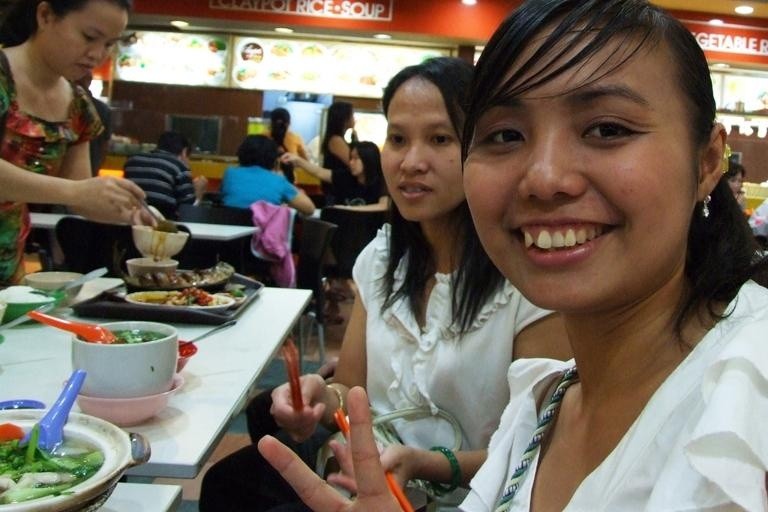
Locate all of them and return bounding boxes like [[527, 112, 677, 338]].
[[0, 398, 152, 512], [177, 340, 198, 374], [125, 257, 179, 277], [71, 321, 180, 396], [24, 271, 85, 307], [0, 290, 64, 325], [131, 224, 190, 258]]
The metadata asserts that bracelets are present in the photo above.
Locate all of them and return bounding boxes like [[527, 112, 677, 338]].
[[328, 384, 345, 425], [429, 445, 464, 493]]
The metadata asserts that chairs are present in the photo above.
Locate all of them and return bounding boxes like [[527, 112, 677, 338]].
[[55, 216, 138, 277], [287, 214, 339, 376], [217, 206, 256, 271]]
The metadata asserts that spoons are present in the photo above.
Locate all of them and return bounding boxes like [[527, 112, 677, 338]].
[[26, 311, 115, 344], [19, 369, 89, 449], [130, 191, 178, 234]]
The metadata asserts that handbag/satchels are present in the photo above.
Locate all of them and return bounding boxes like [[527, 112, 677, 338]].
[[315, 406, 461, 510]]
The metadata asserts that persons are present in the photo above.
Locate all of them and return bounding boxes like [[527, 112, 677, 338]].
[[219, 133, 317, 234], [724, 159, 747, 211], [257, 0, 767, 512], [263, 107, 308, 185], [321, 101, 360, 207], [279, 141, 391, 248], [269, 56, 573, 494], [1, 0, 171, 289], [199, 356, 339, 511], [123, 127, 210, 221]]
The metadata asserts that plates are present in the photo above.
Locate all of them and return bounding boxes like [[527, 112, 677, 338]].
[[62, 372, 186, 428], [68, 268, 266, 324], [124, 290, 236, 314]]
